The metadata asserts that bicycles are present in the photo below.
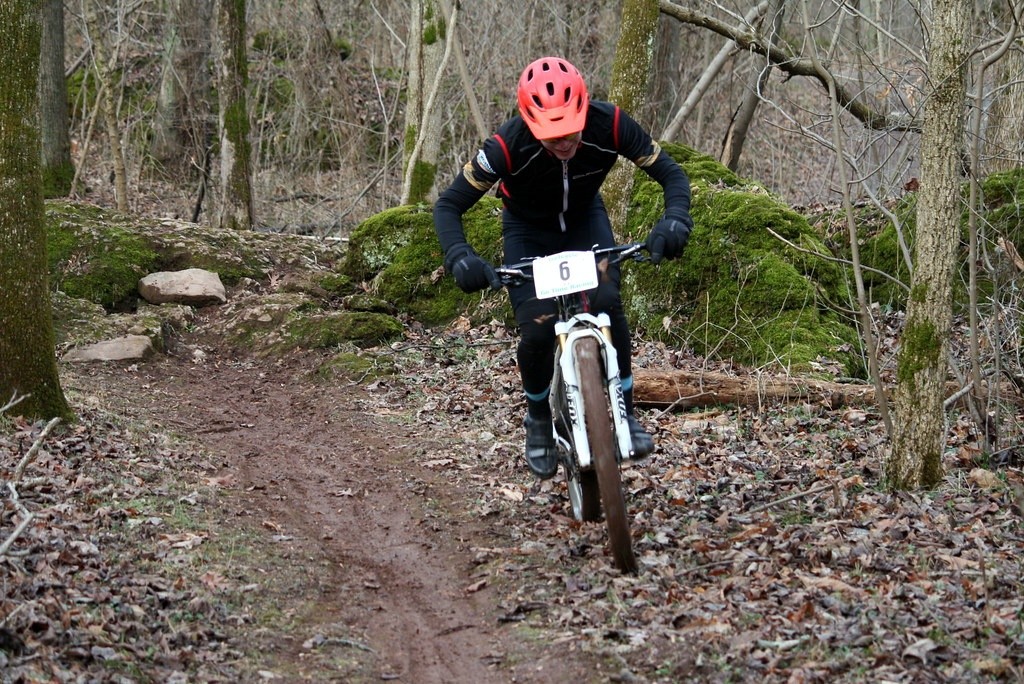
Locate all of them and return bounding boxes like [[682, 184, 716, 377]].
[[491, 242, 639, 576]]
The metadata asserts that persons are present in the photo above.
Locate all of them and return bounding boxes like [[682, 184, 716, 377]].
[[433, 58, 693, 481]]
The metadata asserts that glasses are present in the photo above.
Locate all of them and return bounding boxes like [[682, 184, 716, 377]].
[[543, 131, 579, 143]]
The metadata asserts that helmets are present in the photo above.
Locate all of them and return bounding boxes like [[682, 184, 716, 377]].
[[516, 56, 590, 140]]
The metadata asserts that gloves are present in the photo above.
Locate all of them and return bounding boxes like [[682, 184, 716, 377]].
[[647, 219, 690, 265], [452, 255, 502, 294]]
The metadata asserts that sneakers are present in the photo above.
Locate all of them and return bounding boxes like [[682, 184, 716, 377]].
[[613, 420, 655, 460], [524, 410, 558, 479]]
